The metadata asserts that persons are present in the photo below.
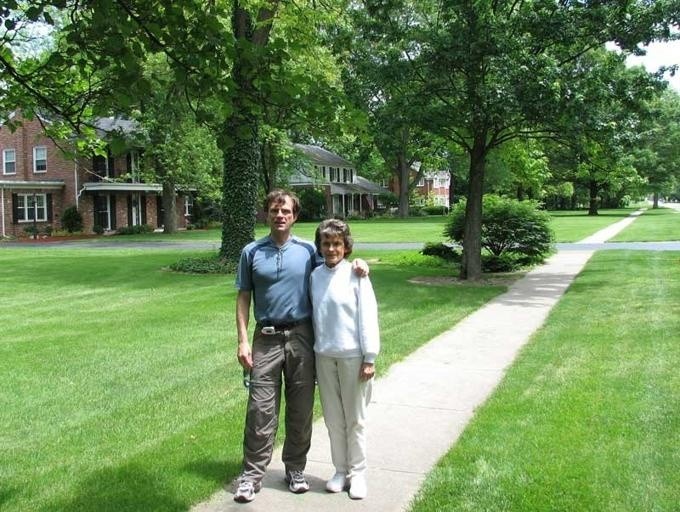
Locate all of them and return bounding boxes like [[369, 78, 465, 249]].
[[234, 187, 370, 503], [308, 217, 379, 500]]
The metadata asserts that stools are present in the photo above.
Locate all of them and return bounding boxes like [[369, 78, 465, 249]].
[[257, 317, 311, 330]]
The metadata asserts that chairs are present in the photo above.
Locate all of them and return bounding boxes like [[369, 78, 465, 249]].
[[234, 481, 261, 502], [349, 478, 367, 499], [285, 470, 309, 493], [326, 473, 350, 493]]
